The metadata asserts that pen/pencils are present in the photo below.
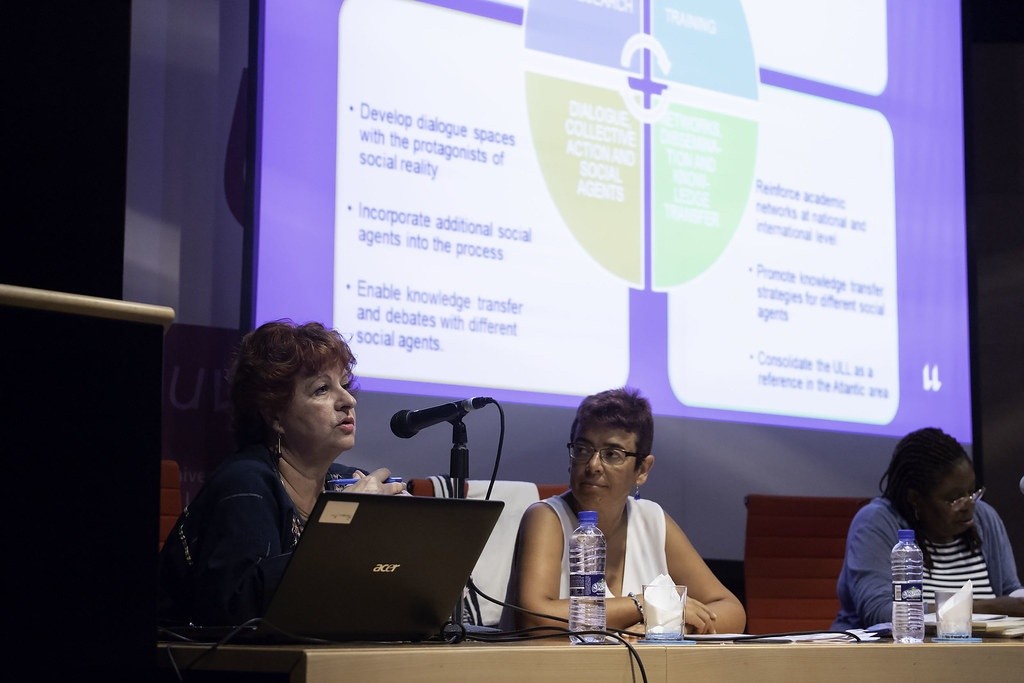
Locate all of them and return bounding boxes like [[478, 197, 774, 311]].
[[327, 477, 402, 486]]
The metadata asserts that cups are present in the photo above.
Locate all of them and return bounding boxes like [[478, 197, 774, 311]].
[[642, 584, 687, 641], [934, 590, 974, 638]]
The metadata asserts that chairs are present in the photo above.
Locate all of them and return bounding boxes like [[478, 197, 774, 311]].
[[408, 477, 572, 629], [743, 493, 883, 637]]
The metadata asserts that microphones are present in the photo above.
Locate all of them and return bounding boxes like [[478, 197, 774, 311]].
[[390, 396, 492, 438]]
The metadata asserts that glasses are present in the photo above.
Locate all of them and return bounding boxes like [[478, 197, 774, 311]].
[[567, 442, 646, 465], [915, 480, 985, 511]]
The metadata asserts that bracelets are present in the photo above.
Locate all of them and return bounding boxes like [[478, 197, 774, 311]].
[[628, 592, 644, 622]]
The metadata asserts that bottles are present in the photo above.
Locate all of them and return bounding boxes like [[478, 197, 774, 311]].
[[890, 530, 925, 643], [568, 510, 606, 645]]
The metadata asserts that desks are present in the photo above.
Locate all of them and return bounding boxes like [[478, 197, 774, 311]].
[[155, 619, 1023, 682]]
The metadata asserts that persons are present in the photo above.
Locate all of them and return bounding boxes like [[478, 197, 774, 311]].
[[498, 390, 748, 642], [160, 319, 414, 640], [826, 427, 1024, 631]]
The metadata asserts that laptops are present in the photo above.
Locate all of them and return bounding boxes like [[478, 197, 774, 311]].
[[172, 491, 504, 646]]
